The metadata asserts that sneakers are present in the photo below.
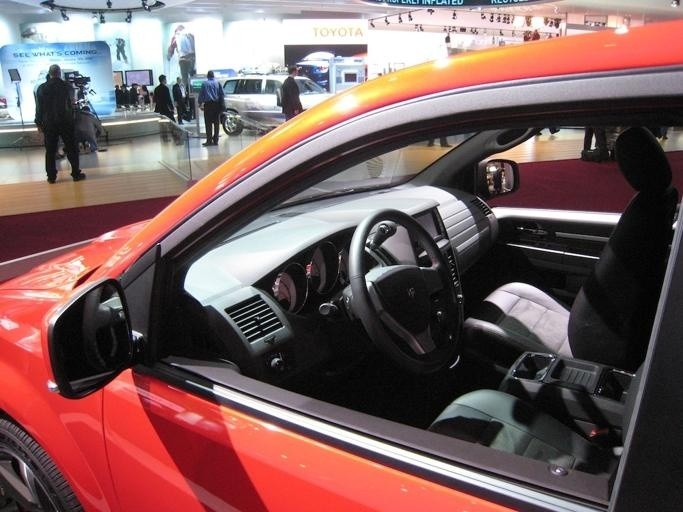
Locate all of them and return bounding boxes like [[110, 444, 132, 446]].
[[428, 143, 454, 146], [55, 153, 67, 160], [46, 175, 58, 184], [202, 140, 219, 147], [70, 169, 85, 182]]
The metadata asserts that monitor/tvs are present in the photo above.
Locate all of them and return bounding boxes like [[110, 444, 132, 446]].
[[125, 69, 153, 86], [113, 71, 123, 86]]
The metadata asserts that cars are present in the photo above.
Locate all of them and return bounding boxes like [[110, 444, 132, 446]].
[[294, 59, 329, 89], [209, 69, 236, 88], [1, 19, 682, 508], [189, 73, 208, 91]]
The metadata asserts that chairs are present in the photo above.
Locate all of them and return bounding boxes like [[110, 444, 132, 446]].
[[462, 121, 678, 374], [422, 361, 643, 477]]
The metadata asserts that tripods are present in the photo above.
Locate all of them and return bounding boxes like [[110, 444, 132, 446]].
[[11, 83, 35, 151]]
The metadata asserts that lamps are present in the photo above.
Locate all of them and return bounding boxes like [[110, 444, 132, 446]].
[[365, 7, 559, 41], [39, 0, 165, 25]]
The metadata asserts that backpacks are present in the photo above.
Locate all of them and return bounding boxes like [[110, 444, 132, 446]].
[[275, 85, 286, 107]]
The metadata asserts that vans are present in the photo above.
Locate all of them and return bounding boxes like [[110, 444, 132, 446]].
[[219, 70, 331, 138]]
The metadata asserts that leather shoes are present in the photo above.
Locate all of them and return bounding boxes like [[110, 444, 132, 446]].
[[536, 129, 560, 135]]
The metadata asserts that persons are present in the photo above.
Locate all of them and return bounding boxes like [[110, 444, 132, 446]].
[[172, 77, 187, 125], [535, 126, 670, 159], [114, 83, 154, 111], [34, 64, 86, 183], [198, 71, 224, 146], [428, 136, 454, 149], [44, 74, 64, 159], [152, 75, 184, 145], [281, 65, 303, 122], [166, 26, 196, 85]]
